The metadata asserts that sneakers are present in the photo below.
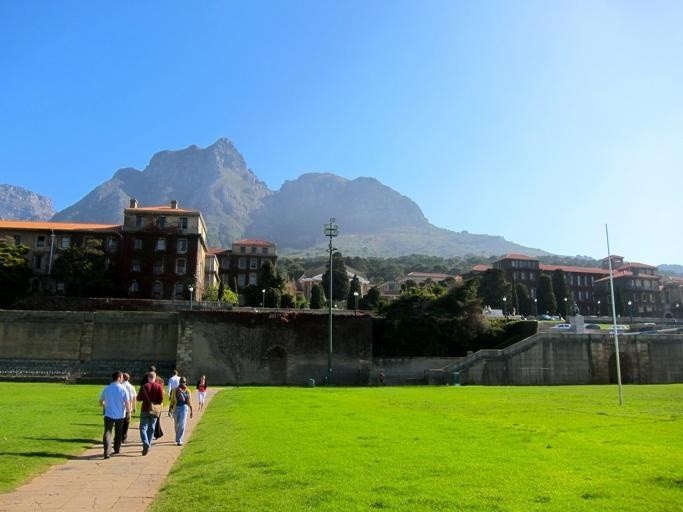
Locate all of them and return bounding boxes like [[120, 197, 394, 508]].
[[178, 442, 182, 446], [142, 445, 150, 455], [105, 454, 110, 458], [123, 440, 127, 443]]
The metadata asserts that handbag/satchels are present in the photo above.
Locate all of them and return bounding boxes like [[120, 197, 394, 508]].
[[149, 403, 162, 417]]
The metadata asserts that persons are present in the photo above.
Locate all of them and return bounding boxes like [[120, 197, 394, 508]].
[[167, 370, 181, 418], [122, 372, 137, 444], [98, 371, 131, 460], [136, 372, 163, 456], [197, 373, 207, 410], [168, 377, 193, 446], [143, 366, 164, 439]]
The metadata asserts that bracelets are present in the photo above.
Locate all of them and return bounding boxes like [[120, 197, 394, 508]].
[[127, 413, 131, 415]]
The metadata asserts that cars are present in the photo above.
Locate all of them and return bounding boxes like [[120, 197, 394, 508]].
[[609, 325, 629, 330], [586, 325, 600, 329], [538, 315, 564, 320], [549, 324, 571, 330]]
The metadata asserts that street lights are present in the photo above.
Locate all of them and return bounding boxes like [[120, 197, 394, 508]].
[[324, 216, 340, 385], [262, 288, 266, 307], [353, 291, 359, 309], [502, 296, 633, 318], [48, 233, 56, 274], [188, 286, 194, 309]]
